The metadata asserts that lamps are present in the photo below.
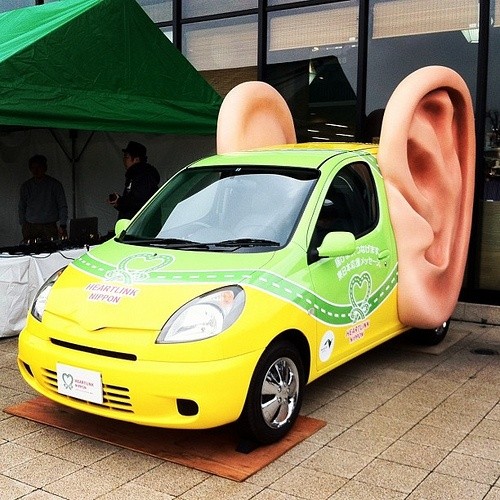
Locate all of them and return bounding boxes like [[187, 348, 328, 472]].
[[460, 14, 493, 44]]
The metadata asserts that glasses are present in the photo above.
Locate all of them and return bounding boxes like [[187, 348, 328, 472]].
[[123, 154, 136, 159]]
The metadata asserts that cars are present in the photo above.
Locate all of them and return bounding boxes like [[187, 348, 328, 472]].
[[16, 140, 453, 443]]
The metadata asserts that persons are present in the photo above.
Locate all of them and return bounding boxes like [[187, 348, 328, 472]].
[[17, 154, 67, 255], [108, 140, 162, 237]]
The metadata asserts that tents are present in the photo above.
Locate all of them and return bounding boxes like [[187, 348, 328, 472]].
[[0, 0, 223, 341]]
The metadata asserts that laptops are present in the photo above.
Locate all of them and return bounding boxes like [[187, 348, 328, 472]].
[[68, 217, 98, 241]]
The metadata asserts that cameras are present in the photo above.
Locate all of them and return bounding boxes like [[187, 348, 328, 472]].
[[109, 194, 122, 204]]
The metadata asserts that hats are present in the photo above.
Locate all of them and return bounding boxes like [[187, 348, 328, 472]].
[[122, 140, 146, 156]]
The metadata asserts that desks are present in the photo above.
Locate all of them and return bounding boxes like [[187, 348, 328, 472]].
[[0, 233, 113, 338]]
